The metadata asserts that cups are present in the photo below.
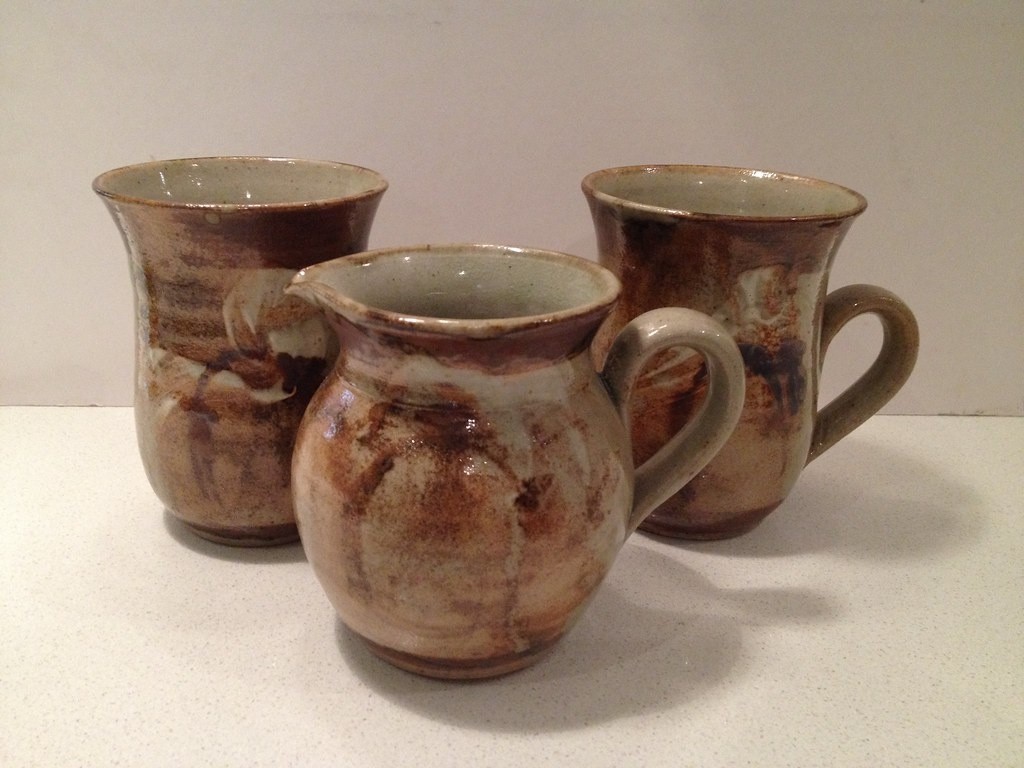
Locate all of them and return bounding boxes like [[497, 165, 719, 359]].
[[92, 155, 920, 549], [288, 242, 746, 682]]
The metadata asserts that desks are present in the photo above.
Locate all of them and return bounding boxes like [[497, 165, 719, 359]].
[[1, 404, 1024, 768]]
